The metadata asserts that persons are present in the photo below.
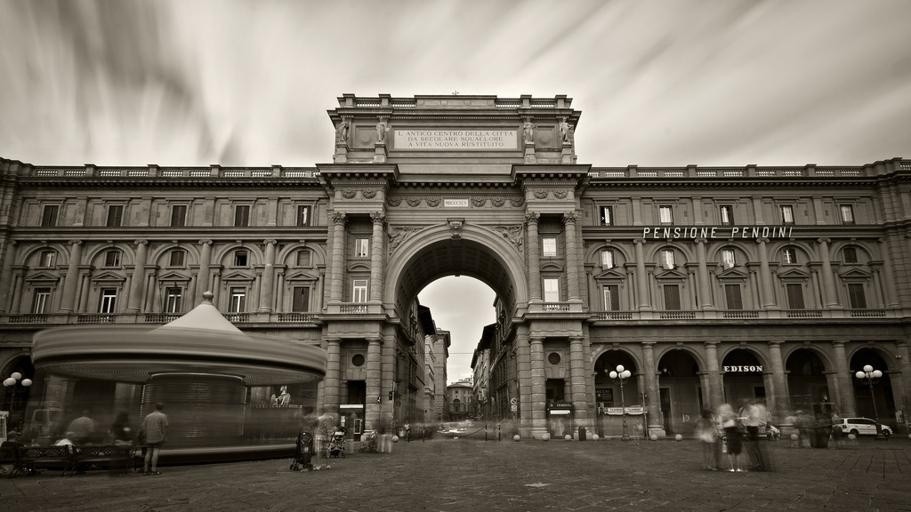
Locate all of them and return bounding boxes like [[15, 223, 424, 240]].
[[290, 406, 347, 471], [54, 408, 95, 454], [142, 402, 168, 476], [1, 430, 24, 462], [108, 409, 133, 445], [30, 438, 40, 446], [694, 398, 768, 473]]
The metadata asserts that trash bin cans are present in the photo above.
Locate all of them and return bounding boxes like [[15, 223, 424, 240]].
[[579, 426, 586, 441]]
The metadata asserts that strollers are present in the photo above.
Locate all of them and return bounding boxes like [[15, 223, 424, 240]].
[[326, 425, 347, 458], [290, 431, 314, 472]]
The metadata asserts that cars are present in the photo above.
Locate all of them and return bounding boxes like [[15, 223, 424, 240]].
[[831, 416, 894, 438], [25, 400, 62, 436]]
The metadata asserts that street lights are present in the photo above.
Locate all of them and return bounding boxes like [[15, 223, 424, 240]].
[[3, 371, 33, 431], [609, 364, 632, 440], [856, 364, 884, 421]]
